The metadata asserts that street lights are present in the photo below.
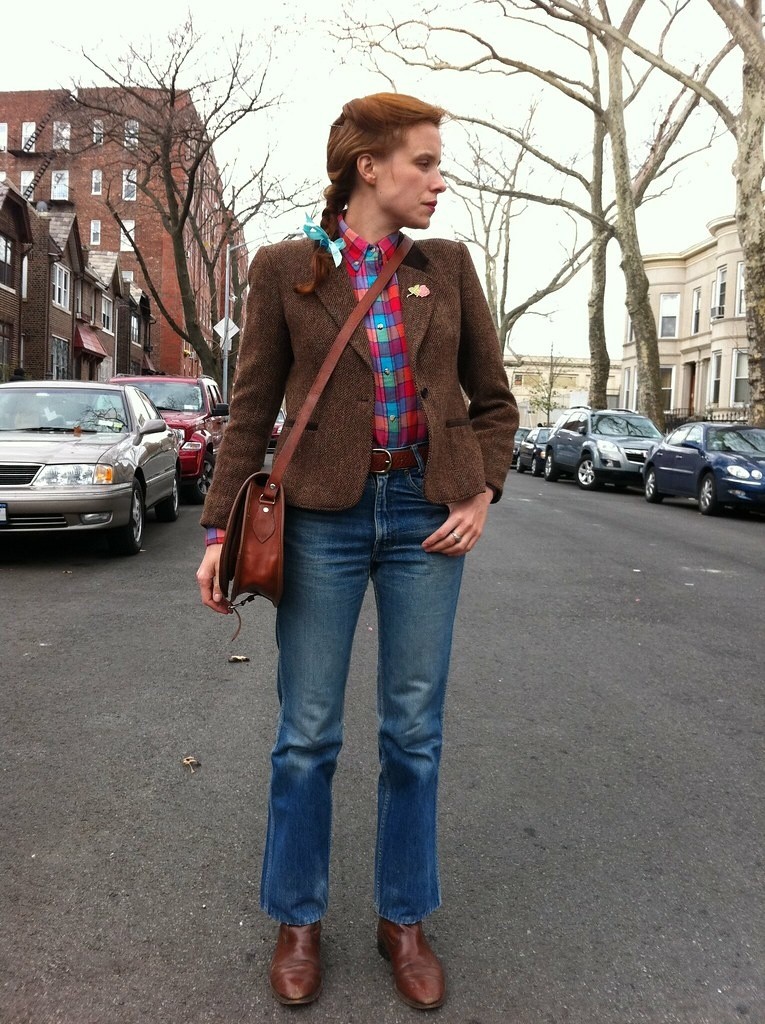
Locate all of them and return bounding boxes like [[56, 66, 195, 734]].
[[223, 232, 305, 403]]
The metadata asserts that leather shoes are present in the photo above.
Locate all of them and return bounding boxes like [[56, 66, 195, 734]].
[[268, 920, 323, 1004], [377, 916, 447, 1009]]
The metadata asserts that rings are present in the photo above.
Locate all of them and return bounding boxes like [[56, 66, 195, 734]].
[[451, 532, 461, 542]]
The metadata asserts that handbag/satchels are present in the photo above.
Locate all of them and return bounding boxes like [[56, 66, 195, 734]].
[[219, 472, 285, 641]]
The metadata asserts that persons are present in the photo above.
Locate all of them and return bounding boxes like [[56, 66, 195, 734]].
[[197, 94, 521, 1011]]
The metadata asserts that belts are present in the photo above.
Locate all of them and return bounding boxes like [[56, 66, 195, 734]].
[[370, 445, 428, 471]]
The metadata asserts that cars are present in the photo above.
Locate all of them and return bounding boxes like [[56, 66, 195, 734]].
[[0, 380, 179, 556], [642, 420, 765, 517], [265, 408, 286, 453], [509, 427, 532, 470], [516, 427, 554, 477]]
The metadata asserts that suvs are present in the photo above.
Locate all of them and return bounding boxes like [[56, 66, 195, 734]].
[[109, 373, 230, 504], [544, 405, 667, 491]]
